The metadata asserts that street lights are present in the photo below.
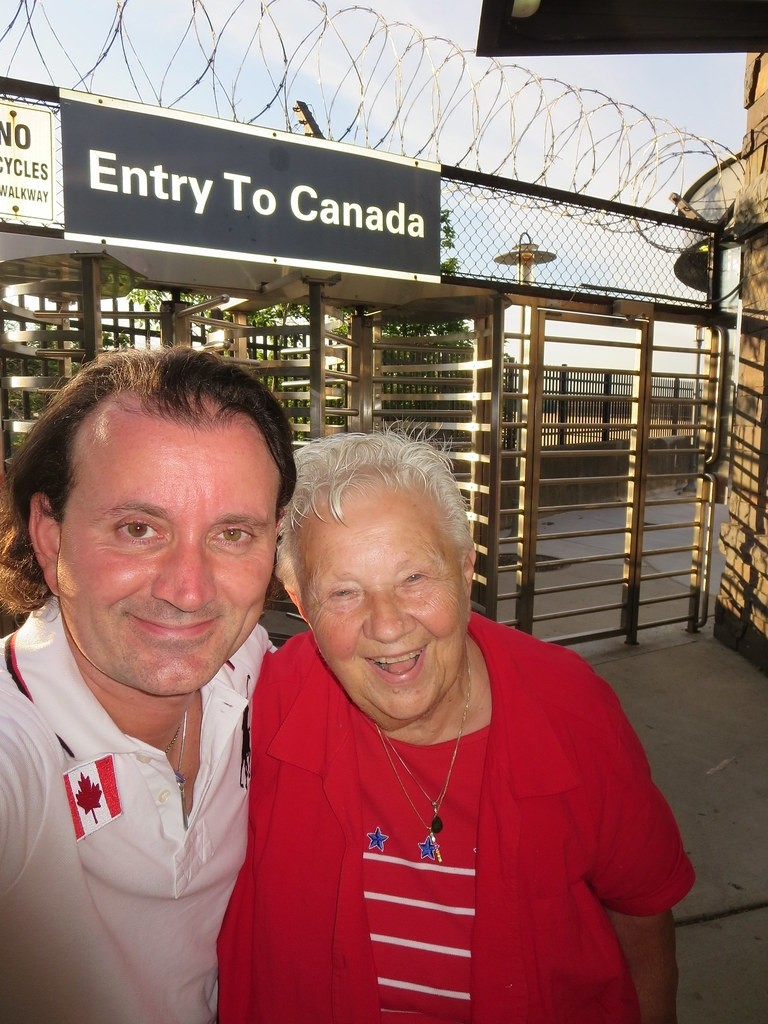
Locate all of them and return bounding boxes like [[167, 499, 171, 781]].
[[493, 231, 556, 538]]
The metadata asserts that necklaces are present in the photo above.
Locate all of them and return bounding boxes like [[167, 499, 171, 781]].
[[159, 711, 189, 830], [374, 647, 471, 864]]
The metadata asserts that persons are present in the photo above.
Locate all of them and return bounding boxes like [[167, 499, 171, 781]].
[[216, 429, 696, 1024], [0, 350, 297, 1023]]
